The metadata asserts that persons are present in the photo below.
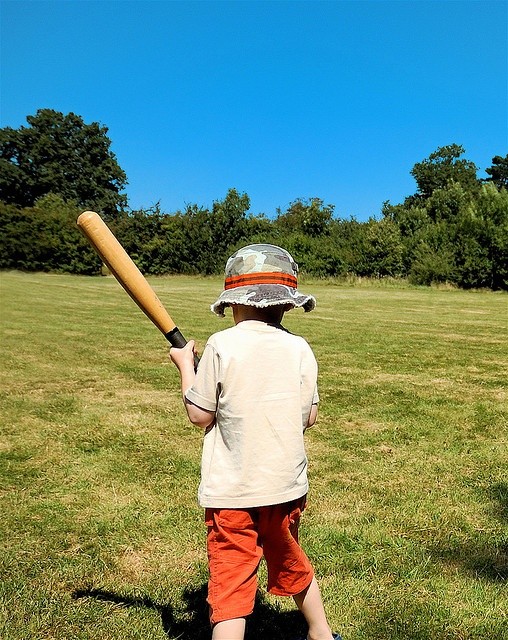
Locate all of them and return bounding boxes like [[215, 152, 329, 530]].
[[170, 243, 343, 640]]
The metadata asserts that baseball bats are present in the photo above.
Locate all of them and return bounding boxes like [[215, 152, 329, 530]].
[[77, 211, 200, 375]]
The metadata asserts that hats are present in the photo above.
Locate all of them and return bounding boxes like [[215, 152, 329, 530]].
[[210, 244, 316, 317]]
